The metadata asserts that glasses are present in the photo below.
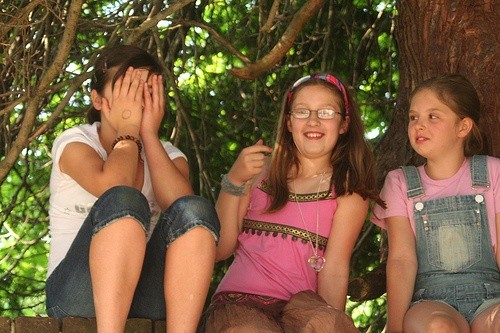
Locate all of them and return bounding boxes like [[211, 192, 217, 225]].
[[288, 107, 347, 119]]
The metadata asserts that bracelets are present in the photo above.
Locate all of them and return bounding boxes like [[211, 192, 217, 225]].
[[218, 174, 247, 196], [112, 135, 143, 153]]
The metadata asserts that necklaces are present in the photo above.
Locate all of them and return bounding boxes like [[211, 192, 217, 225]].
[[292, 171, 327, 272]]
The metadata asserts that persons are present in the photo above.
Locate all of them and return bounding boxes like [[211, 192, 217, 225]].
[[370, 74, 500, 333], [46, 45, 221, 333], [206, 73, 388, 333]]
